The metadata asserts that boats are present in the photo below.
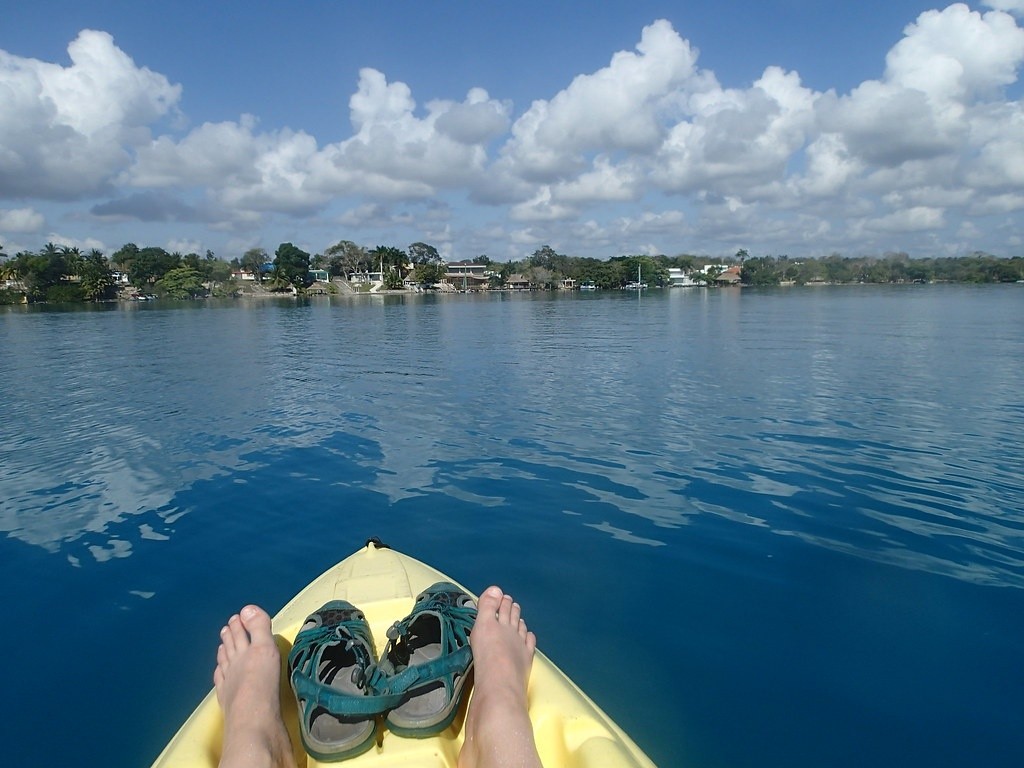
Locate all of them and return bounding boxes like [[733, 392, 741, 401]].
[[580, 280, 600, 289], [625, 281, 648, 288]]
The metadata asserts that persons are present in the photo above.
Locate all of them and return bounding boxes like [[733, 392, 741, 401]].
[[214, 585, 543, 768]]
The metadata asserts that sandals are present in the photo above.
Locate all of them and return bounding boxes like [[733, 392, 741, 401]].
[[288, 596, 407, 763], [369, 581, 481, 739]]
